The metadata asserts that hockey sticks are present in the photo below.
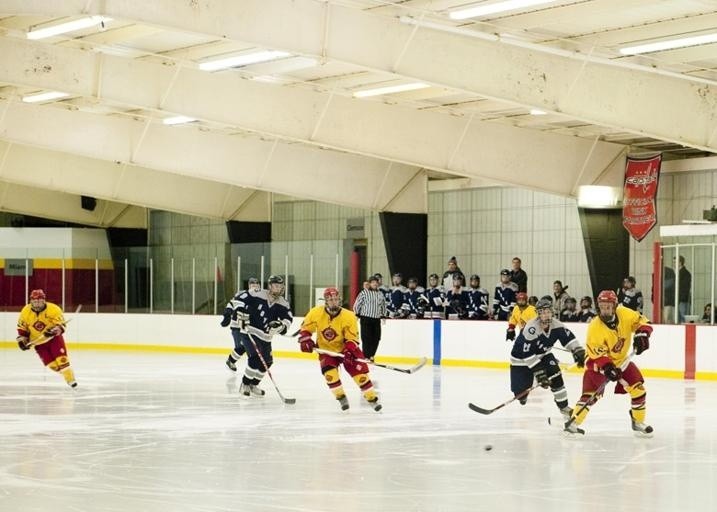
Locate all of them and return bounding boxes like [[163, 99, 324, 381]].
[[548, 350, 637, 424], [27, 302, 83, 349], [313, 346, 426, 374], [468, 359, 577, 415], [246, 327, 297, 406]]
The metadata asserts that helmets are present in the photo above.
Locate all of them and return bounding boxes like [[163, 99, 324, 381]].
[[427, 256, 479, 282], [500, 269, 510, 276], [368, 273, 418, 286], [622, 276, 635, 286], [323, 287, 342, 311], [29, 288, 46, 311], [563, 296, 576, 304], [515, 293, 527, 307], [580, 296, 592, 304], [248, 278, 261, 292], [267, 275, 284, 296], [528, 295, 553, 323], [596, 290, 618, 320]]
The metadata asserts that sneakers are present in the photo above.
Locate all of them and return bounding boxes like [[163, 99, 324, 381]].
[[631, 420, 652, 434], [239, 382, 250, 397], [68, 379, 77, 387], [562, 418, 585, 434], [371, 402, 382, 411], [559, 405, 573, 415], [225, 359, 236, 371], [519, 391, 529, 404], [339, 396, 348, 410], [248, 383, 265, 396]]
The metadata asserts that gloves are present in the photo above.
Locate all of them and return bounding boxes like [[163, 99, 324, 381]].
[[16, 335, 29, 350], [601, 362, 622, 380], [532, 368, 552, 389], [505, 328, 515, 341], [43, 322, 65, 337], [632, 329, 648, 354], [572, 349, 585, 367], [220, 308, 232, 326], [268, 320, 287, 335], [337, 341, 364, 371], [298, 330, 318, 352]]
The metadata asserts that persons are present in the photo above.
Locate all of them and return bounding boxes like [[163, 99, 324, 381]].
[[510, 296, 589, 417], [663, 265, 675, 323], [218, 276, 263, 371], [15, 289, 78, 389], [673, 256, 691, 323], [350, 275, 386, 360], [562, 287, 656, 436], [702, 302, 717, 325], [298, 284, 385, 412], [505, 291, 540, 342], [357, 257, 644, 325], [240, 274, 298, 397]]
